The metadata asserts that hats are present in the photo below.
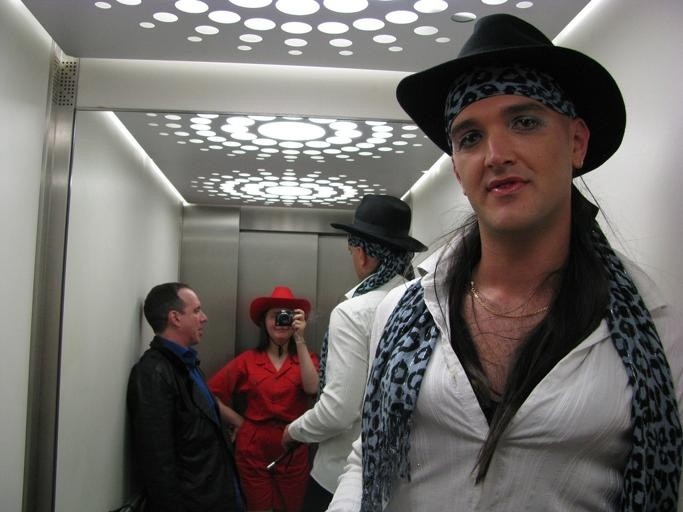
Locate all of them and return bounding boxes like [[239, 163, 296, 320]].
[[249, 286, 311, 325], [395, 13, 627, 180], [330, 195, 428, 251]]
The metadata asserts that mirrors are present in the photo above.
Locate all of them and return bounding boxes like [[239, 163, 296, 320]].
[[53, 105, 474, 510]]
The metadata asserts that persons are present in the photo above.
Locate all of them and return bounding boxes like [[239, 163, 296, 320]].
[[205, 287, 318, 512], [323, 14, 682, 512], [281, 194, 428, 512], [127, 283, 247, 511]]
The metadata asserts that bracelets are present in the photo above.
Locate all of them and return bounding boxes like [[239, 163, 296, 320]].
[[295, 339, 304, 344]]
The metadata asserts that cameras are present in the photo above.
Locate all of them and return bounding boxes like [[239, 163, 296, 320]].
[[275, 310, 296, 326]]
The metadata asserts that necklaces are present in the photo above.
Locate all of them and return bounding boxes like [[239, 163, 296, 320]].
[[468, 279, 550, 320]]
